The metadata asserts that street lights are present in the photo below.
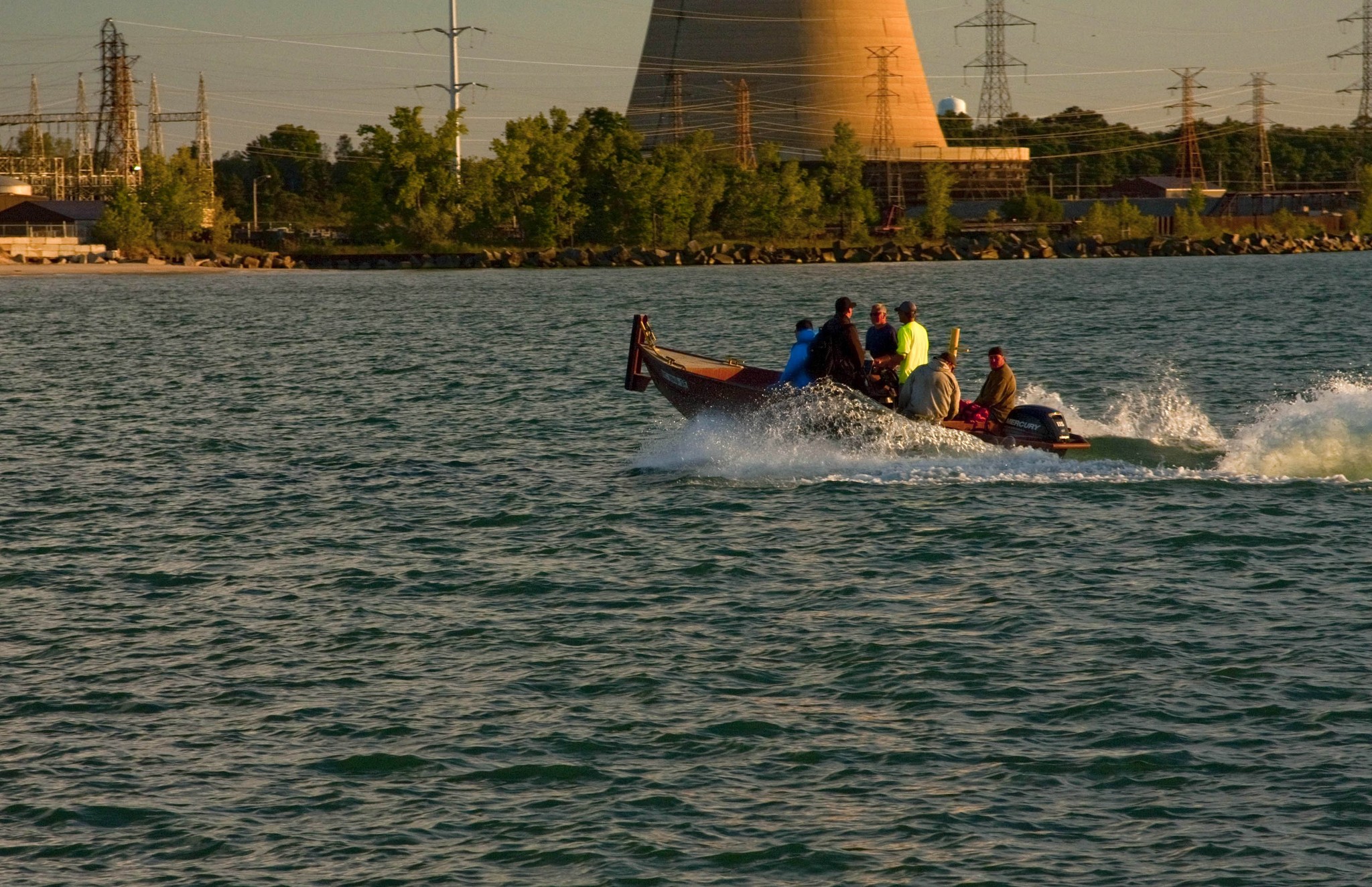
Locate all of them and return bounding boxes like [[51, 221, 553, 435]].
[[253, 175, 271, 229]]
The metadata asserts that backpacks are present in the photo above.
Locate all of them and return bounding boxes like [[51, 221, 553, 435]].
[[810, 323, 853, 378]]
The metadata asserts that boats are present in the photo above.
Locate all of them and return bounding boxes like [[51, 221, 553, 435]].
[[624, 312, 1091, 461]]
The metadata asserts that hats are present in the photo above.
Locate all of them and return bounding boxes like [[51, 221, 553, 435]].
[[988, 347, 1003, 355], [894, 301, 917, 312], [933, 352, 956, 365], [835, 297, 856, 309]]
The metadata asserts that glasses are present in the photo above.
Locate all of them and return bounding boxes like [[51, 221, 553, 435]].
[[870, 312, 883, 316], [794, 330, 797, 333]]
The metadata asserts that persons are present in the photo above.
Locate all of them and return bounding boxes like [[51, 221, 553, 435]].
[[865, 304, 897, 380], [767, 320, 817, 391], [889, 301, 929, 399], [897, 351, 960, 424], [973, 347, 1017, 424], [808, 296, 864, 386]]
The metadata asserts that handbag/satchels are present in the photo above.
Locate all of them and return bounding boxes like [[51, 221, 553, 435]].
[[866, 365, 898, 398], [959, 399, 989, 424]]
